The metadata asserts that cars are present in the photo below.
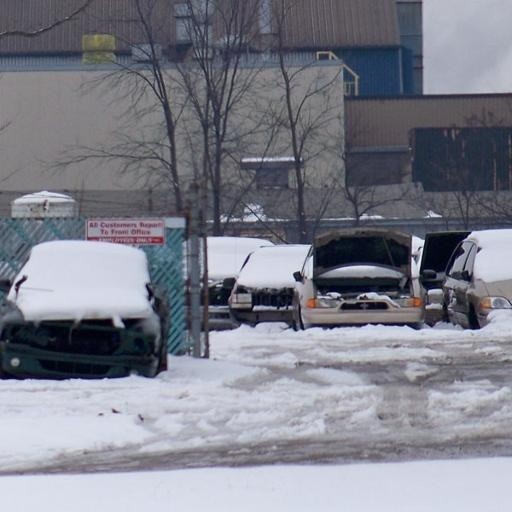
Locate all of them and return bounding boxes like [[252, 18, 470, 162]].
[[230, 242, 312, 334], [292, 226, 439, 332], [179, 234, 276, 336], [418, 228, 473, 331], [1, 239, 168, 383], [441, 228, 512, 330]]
